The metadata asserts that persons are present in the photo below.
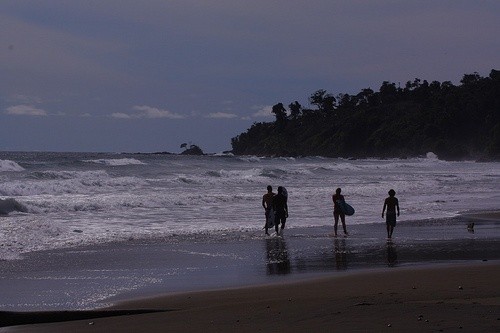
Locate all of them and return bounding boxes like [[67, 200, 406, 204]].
[[382, 190, 400, 238], [262, 185, 277, 237], [274, 186, 286, 236], [332, 188, 349, 237]]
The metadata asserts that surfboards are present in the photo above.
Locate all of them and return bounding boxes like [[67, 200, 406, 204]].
[[267, 187, 288, 227], [344, 202, 355, 216]]
